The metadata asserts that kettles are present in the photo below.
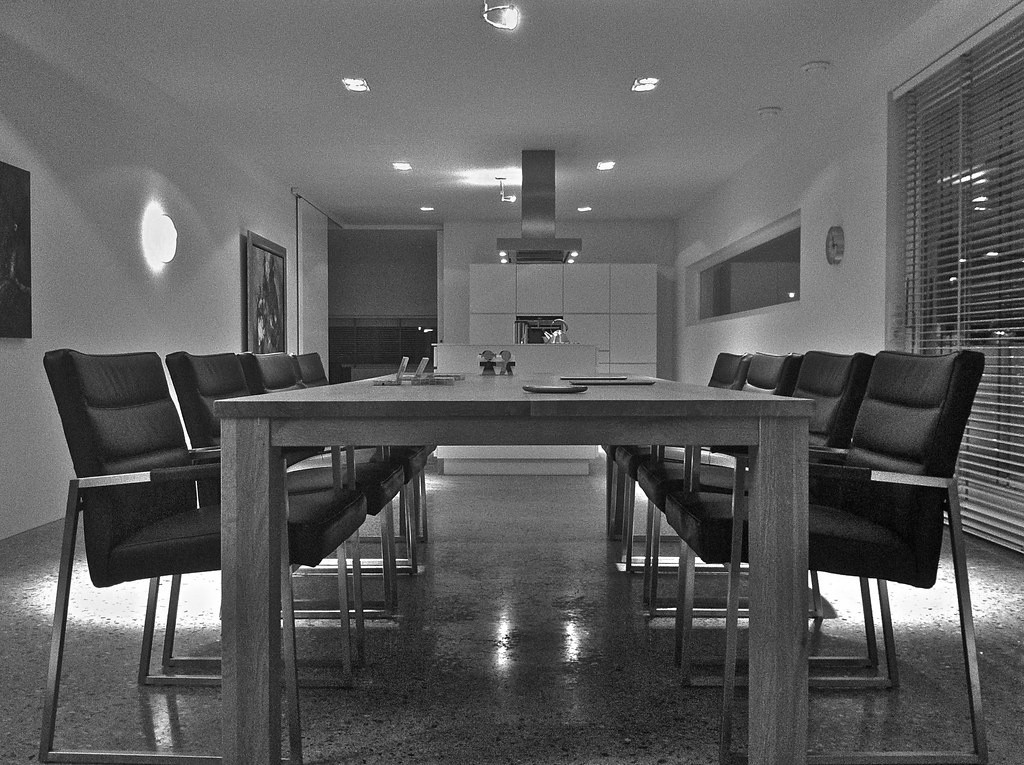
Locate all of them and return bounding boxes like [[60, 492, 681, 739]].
[[542, 319, 569, 344]]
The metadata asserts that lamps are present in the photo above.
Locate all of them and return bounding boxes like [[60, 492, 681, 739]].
[[483, 1, 519, 31], [495, 177, 517, 203]]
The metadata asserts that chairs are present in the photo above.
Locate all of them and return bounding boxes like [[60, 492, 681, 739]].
[[289, 353, 437, 567], [615, 352, 805, 606], [601, 352, 756, 563], [239, 352, 428, 620], [665, 352, 991, 765], [158, 351, 406, 673], [635, 350, 882, 672], [37, 348, 368, 765]]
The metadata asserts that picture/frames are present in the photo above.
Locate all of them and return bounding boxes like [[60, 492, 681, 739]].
[[0, 161, 33, 340], [247, 229, 287, 354]]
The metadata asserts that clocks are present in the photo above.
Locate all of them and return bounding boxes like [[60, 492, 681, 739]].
[[825, 226, 845, 265]]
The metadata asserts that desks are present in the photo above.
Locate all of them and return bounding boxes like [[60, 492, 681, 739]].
[[210, 373, 816, 764]]
